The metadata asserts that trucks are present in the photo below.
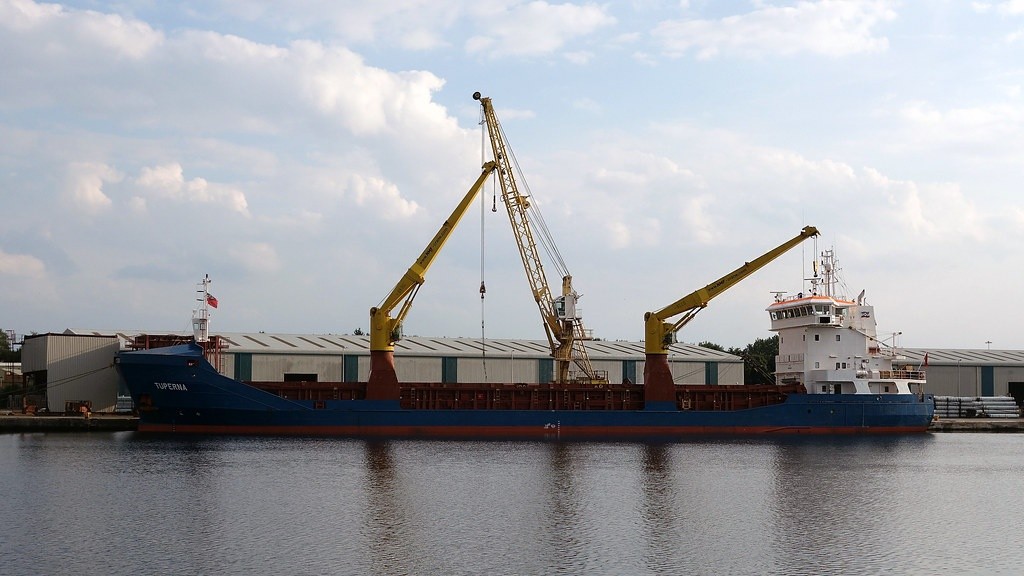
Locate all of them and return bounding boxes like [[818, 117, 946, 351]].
[[116, 395, 133, 415]]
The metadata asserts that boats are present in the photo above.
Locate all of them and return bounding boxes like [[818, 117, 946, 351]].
[[110, 249, 935, 442]]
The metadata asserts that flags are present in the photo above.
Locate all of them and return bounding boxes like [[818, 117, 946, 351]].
[[924, 353, 929, 369], [206, 292, 218, 309]]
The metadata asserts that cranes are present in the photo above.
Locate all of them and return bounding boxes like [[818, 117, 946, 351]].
[[645, 226, 822, 399], [471, 92, 611, 385], [366, 162, 496, 401]]
